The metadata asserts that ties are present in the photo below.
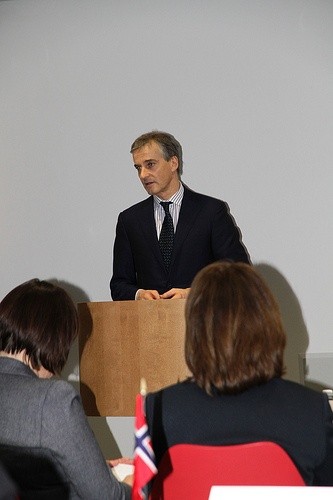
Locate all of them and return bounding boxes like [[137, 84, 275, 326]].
[[158, 201, 174, 269]]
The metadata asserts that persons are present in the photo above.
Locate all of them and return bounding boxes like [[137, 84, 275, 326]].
[[145, 263, 333, 500], [0, 277, 132, 499], [110, 131, 252, 301]]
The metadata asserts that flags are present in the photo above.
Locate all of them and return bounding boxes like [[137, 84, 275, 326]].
[[132, 393, 159, 499]]
